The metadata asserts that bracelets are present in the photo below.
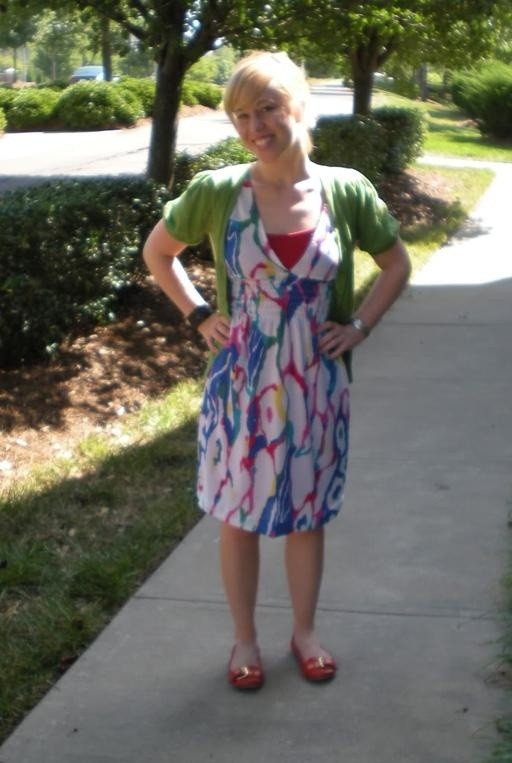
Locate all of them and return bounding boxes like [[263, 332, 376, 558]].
[[184, 302, 216, 330]]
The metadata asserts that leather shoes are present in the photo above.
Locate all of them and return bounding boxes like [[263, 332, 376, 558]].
[[291, 636, 336, 682], [229, 644, 263, 689]]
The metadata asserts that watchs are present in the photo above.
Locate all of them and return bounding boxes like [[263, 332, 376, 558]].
[[349, 316, 370, 340]]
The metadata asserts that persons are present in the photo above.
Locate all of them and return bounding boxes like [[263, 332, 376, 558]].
[[137, 44, 413, 693]]
